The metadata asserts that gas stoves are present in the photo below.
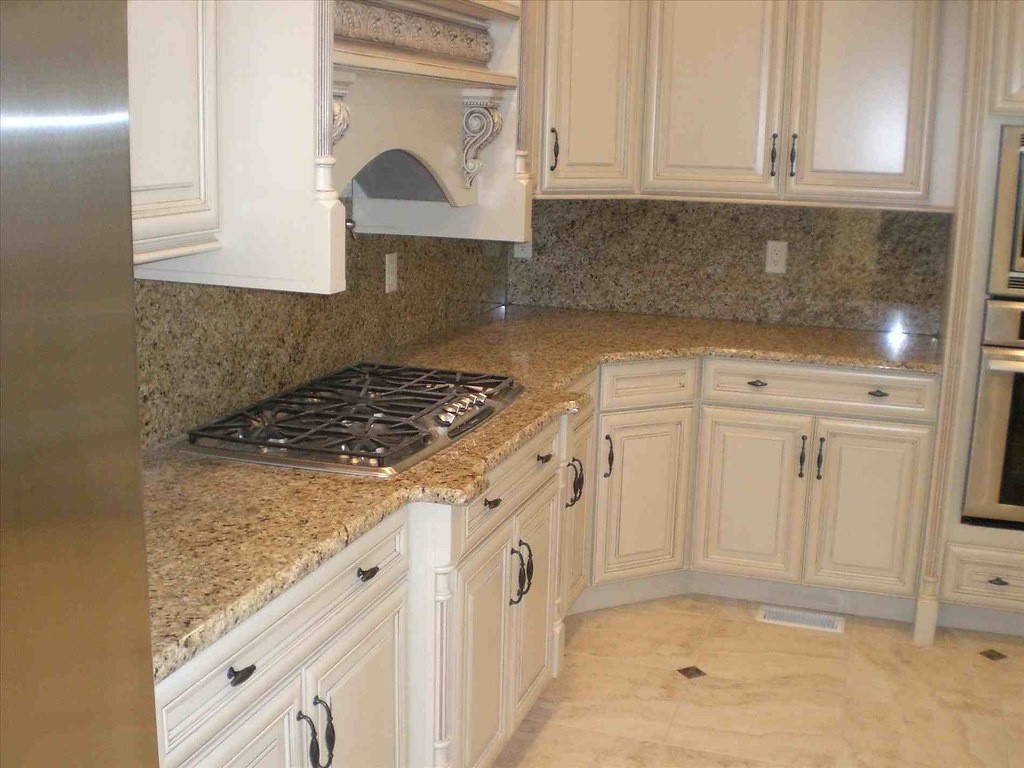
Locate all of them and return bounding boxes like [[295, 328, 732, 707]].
[[190, 364, 523, 476]]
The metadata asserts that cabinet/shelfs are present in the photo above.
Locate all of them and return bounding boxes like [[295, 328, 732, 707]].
[[559, 367, 600, 622], [691, 355, 943, 622], [519, 0, 650, 200], [154, 505, 408, 768], [126, 0, 222, 266], [640, 0, 973, 214], [593, 355, 701, 610], [407, 415, 563, 768]]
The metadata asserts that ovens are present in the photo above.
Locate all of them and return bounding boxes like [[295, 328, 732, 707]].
[[985, 126, 1024, 299], [962, 300, 1024, 524]]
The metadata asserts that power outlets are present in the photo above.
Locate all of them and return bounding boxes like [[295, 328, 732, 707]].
[[386, 252, 398, 294], [513, 229, 532, 258], [765, 240, 789, 273]]
